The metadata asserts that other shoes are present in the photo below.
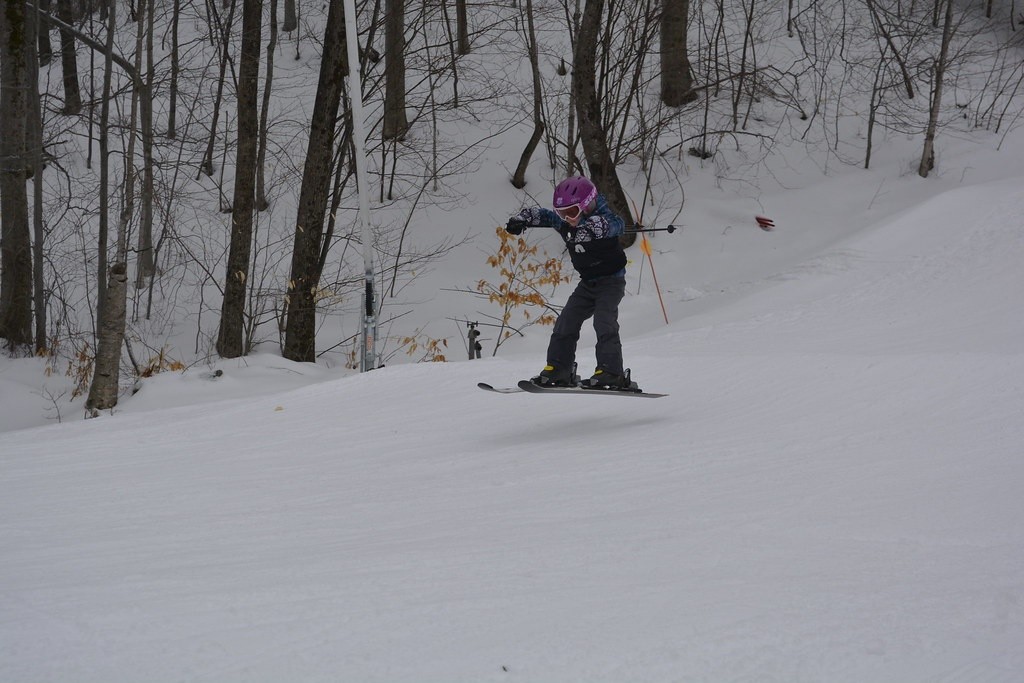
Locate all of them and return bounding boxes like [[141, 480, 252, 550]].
[[542, 364, 572, 381], [592, 370, 626, 384]]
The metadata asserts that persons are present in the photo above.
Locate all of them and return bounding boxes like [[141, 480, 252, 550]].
[[505, 176, 630, 394]]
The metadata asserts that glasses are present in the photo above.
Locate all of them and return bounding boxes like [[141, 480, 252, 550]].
[[555, 187, 598, 220]]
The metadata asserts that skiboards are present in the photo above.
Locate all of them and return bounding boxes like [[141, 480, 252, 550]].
[[477, 380, 669, 398]]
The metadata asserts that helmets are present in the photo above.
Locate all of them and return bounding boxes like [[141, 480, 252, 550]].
[[553, 176, 595, 208]]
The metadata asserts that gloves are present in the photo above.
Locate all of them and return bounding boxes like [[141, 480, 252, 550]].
[[506, 217, 526, 236]]
